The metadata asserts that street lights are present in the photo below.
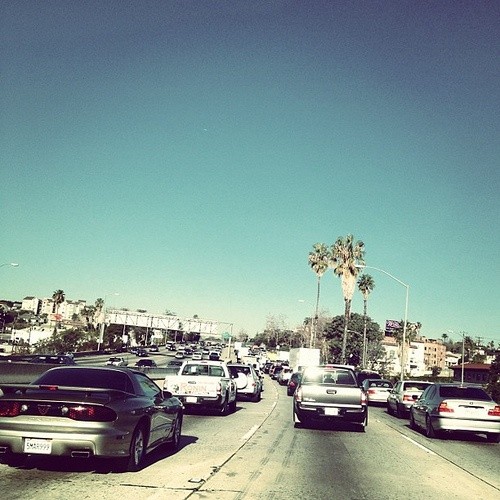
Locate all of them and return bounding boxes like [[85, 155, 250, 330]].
[[103, 292, 120, 321], [298, 300, 316, 349], [448, 330, 464, 384], [355, 265, 409, 381], [347, 330, 368, 366]]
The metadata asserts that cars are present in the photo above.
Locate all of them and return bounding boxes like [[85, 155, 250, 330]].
[[354, 371, 382, 387], [0, 366, 185, 473], [409, 383, 500, 443], [387, 381, 436, 419], [361, 379, 394, 406], [0, 341, 299, 396], [293, 365, 368, 431]]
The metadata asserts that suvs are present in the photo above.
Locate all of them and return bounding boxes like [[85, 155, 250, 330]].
[[226, 365, 261, 402]]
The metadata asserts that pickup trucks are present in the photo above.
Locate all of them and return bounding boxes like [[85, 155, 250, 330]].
[[163, 360, 239, 416]]
